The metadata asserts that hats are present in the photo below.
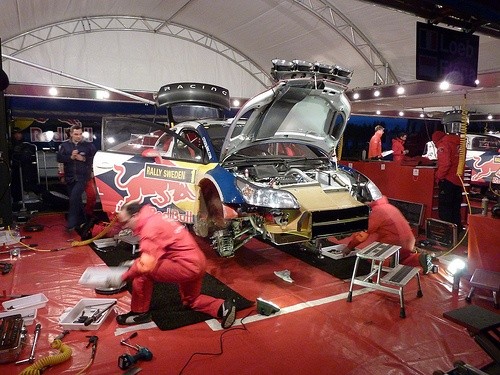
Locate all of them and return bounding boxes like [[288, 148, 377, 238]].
[[432, 130, 445, 144], [13, 127, 23, 135]]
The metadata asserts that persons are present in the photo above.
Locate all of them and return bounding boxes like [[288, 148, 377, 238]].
[[369, 125, 385, 160], [58, 125, 97, 232], [342, 195, 433, 274], [392, 131, 409, 161], [116, 202, 237, 330], [11, 127, 34, 211], [432, 131, 467, 244]]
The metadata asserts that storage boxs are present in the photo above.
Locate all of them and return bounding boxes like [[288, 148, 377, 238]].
[[319, 244, 362, 260], [388, 198, 424, 226], [58, 297, 117, 330], [0, 320, 27, 363], [415, 218, 458, 257], [0, 293, 49, 325], [79, 265, 129, 288]]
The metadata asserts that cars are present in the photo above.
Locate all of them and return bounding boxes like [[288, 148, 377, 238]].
[[93, 57, 383, 259], [38, 149, 65, 178]]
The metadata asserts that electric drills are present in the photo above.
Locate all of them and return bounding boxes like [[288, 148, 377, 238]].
[[118, 343, 153, 369]]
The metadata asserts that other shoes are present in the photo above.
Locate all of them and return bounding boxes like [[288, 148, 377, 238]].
[[418, 253, 433, 274]]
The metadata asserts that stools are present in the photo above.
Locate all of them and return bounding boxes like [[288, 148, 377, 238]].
[[465, 267, 500, 309]]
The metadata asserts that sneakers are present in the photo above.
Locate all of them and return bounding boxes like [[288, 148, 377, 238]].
[[217, 300, 237, 329], [116, 311, 152, 325]]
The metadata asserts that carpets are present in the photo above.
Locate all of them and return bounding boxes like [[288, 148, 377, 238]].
[[250, 232, 371, 279], [63, 212, 256, 331]]
